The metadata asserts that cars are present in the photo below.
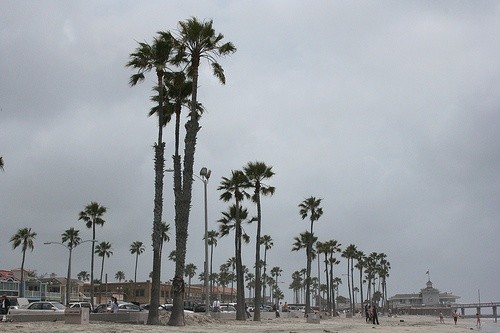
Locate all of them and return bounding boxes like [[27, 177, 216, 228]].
[[22, 300, 306, 314]]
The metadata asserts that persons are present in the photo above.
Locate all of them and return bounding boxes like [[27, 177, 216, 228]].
[[245, 303, 252, 318], [453, 310, 458, 325], [273, 302, 288, 317], [439, 310, 446, 324], [475, 313, 481, 326], [0, 295, 11, 321], [364, 305, 379, 325], [107, 296, 118, 314], [212, 298, 220, 312]]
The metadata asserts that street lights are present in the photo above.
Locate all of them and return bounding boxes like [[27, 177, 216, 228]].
[[165, 167, 212, 313], [43, 240, 99, 308]]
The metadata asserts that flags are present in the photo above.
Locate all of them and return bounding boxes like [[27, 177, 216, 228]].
[[426, 271, 429, 274]]
